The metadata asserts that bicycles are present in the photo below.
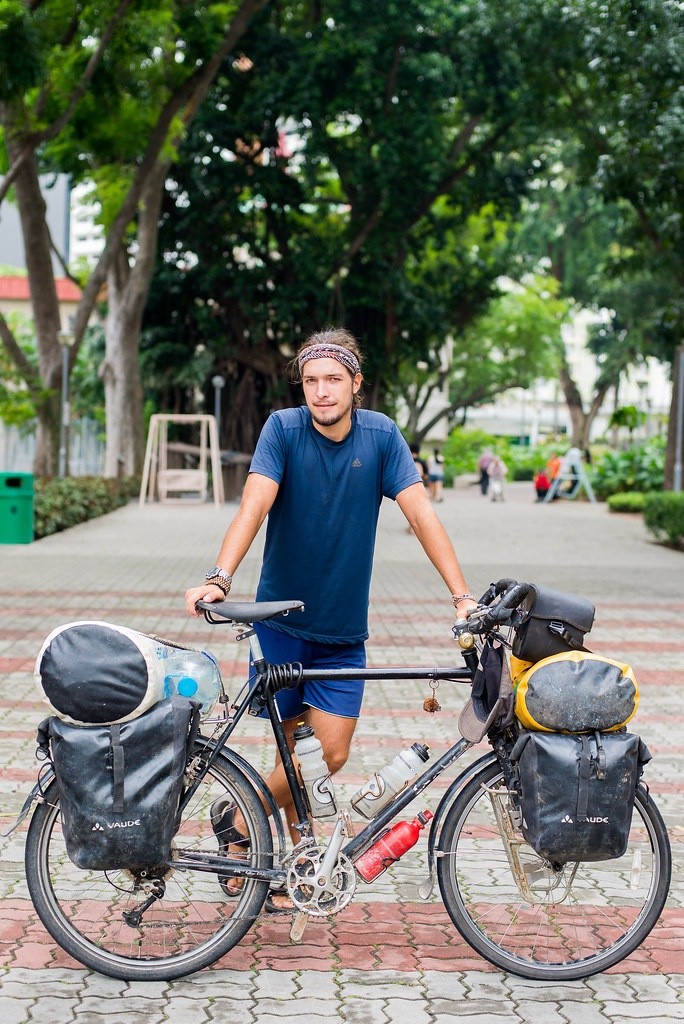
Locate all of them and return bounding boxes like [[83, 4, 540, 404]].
[[0, 575, 672, 980]]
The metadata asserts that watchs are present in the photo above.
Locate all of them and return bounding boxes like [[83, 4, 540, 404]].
[[206, 566, 233, 584]]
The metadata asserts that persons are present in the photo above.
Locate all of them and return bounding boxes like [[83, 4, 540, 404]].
[[532, 452, 565, 501], [478, 446, 508, 501], [426, 447, 445, 503], [407, 443, 429, 533], [185, 326, 487, 912]]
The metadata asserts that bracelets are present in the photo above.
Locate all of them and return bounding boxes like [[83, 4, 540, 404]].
[[451, 594, 475, 607], [204, 576, 230, 596]]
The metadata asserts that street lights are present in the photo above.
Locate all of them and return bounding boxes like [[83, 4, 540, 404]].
[[214, 376, 225, 437], [56, 330, 77, 479]]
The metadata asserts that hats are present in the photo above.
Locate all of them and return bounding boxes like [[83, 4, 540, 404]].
[[454, 631, 516, 747]]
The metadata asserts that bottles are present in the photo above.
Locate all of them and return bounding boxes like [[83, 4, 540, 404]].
[[354, 810, 433, 883], [162, 650, 221, 708], [351, 743, 430, 818], [293, 721, 338, 818]]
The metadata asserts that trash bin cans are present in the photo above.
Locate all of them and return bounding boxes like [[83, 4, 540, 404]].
[[0, 472, 35, 543]]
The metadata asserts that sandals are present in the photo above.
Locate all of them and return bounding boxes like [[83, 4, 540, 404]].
[[262, 883, 306, 915], [210, 799, 249, 898]]
